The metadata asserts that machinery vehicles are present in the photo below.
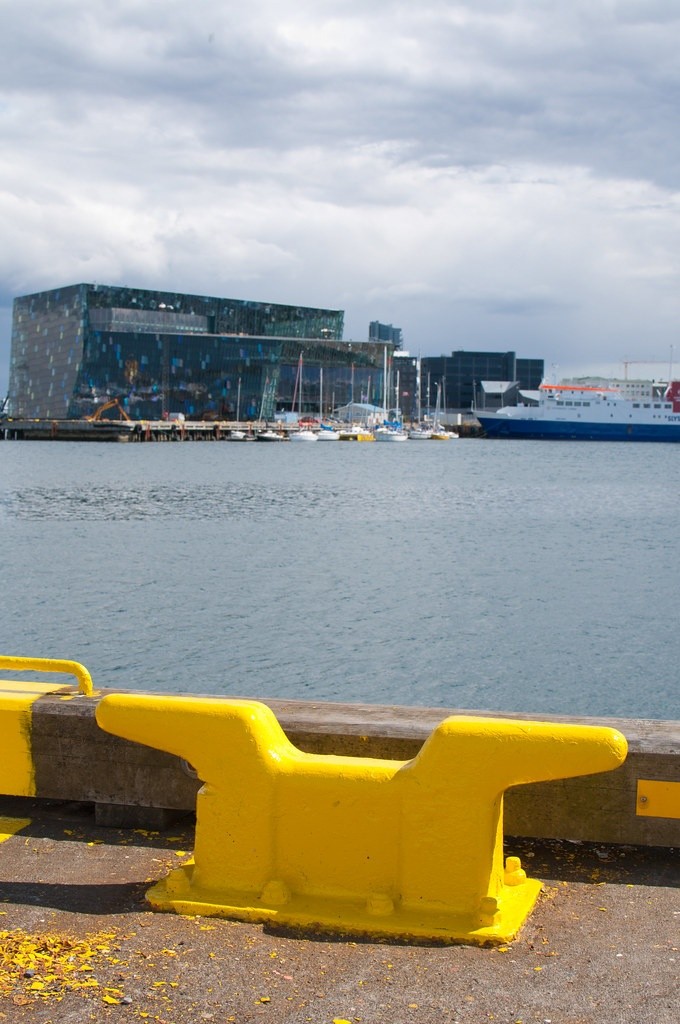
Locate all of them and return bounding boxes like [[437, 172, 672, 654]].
[[83, 399, 130, 421]]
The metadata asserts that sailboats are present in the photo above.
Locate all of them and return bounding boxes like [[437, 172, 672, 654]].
[[292, 351, 461, 441]]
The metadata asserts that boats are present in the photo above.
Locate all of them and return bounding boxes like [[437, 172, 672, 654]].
[[257, 429, 289, 442], [471, 386, 679, 447], [224, 431, 256, 442]]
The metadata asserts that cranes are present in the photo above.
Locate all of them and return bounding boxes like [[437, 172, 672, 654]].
[[624, 360, 678, 382]]
